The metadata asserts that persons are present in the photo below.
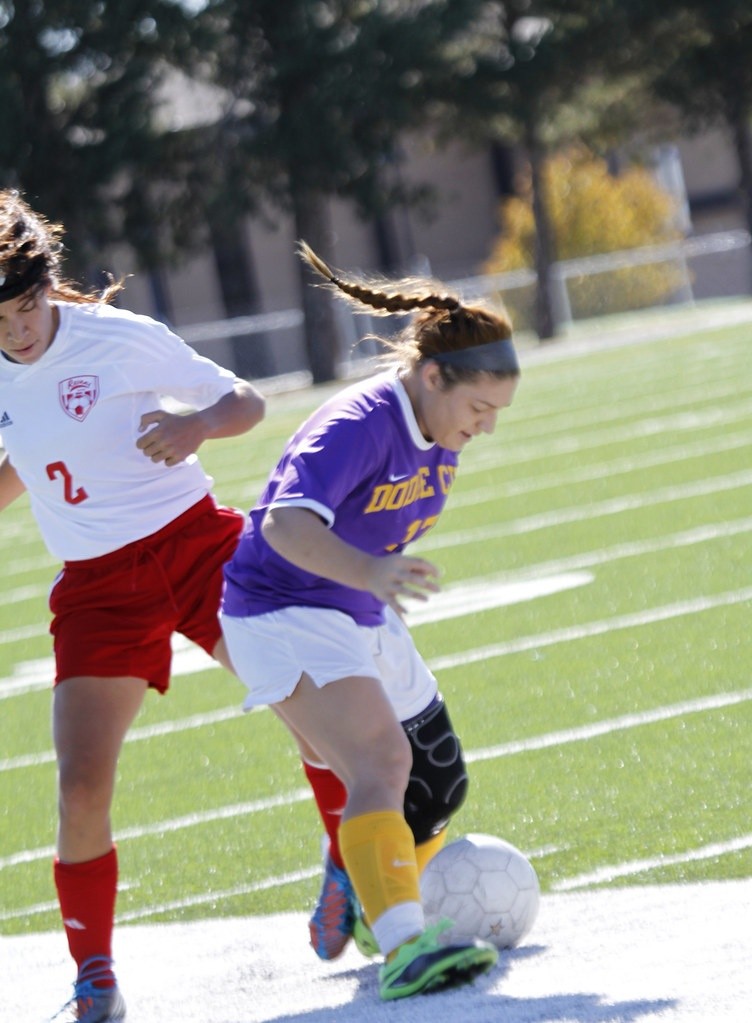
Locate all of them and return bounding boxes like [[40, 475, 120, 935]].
[[217, 242, 519, 1002], [0, 191, 357, 1023]]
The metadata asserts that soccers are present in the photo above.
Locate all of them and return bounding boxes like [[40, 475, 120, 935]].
[[419, 834, 541, 950]]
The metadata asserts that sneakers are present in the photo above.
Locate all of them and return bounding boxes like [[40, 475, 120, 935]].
[[309, 835, 361, 960], [351, 888, 384, 957], [375, 918, 500, 1000], [74, 956, 124, 1023]]
